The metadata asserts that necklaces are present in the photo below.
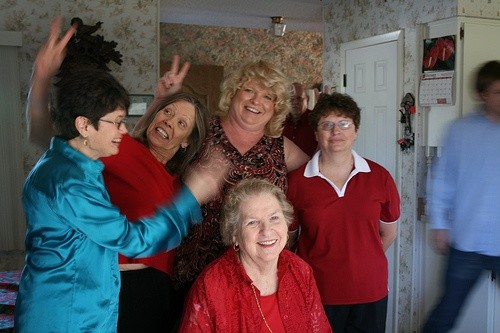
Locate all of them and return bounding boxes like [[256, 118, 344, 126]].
[[251, 283, 274, 333]]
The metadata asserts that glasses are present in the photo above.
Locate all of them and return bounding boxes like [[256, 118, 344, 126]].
[[99, 118, 124, 130], [314, 120, 356, 130]]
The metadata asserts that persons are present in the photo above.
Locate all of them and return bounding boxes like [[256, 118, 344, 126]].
[[178, 177, 332, 333], [14, 68, 227, 333], [281, 82, 317, 159], [286, 93, 401, 333], [131, 54, 329, 333], [419, 59, 500, 333], [24, 15, 208, 333]]
